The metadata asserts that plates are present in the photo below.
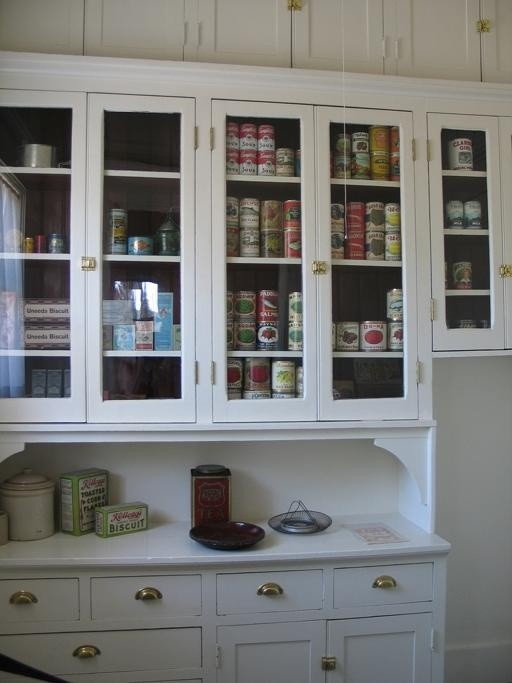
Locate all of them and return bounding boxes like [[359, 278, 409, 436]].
[[188, 521, 265, 551], [268, 509, 333, 535]]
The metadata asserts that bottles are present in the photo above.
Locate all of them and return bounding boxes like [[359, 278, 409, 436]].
[[191, 465, 231, 528]]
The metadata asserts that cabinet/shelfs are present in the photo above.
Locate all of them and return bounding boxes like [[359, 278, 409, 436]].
[[397, 0, 511, 83], [199, 0, 384, 78], [0, 515, 452, 681], [428, 112, 512, 353], [206, 98, 421, 424], [0, 88, 197, 425], [1, 0, 185, 60]]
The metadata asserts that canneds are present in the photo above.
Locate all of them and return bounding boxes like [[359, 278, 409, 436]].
[[464, 201, 482, 229], [345, 233, 364, 260], [366, 232, 385, 261], [447, 135, 473, 171], [226, 122, 239, 150], [334, 133, 351, 155], [351, 133, 369, 152], [48, 233, 65, 253], [288, 322, 304, 351], [23, 237, 33, 252], [390, 153, 400, 180], [260, 200, 282, 229], [104, 209, 128, 255], [244, 391, 271, 398], [332, 322, 336, 350], [284, 229, 302, 258], [331, 231, 344, 258], [366, 202, 384, 231], [273, 392, 296, 398], [239, 123, 257, 151], [156, 207, 179, 255], [239, 151, 258, 175], [258, 124, 275, 151], [385, 203, 400, 231], [360, 321, 388, 351], [227, 226, 239, 255], [351, 154, 370, 180], [244, 357, 270, 390], [257, 288, 278, 321], [296, 366, 303, 393], [226, 151, 239, 174], [239, 197, 260, 227], [227, 358, 243, 388], [234, 321, 256, 350], [226, 290, 233, 322], [331, 203, 345, 232], [333, 156, 352, 179], [261, 230, 284, 257], [387, 288, 404, 321], [235, 291, 257, 322], [371, 153, 391, 181], [288, 291, 303, 322], [336, 322, 359, 351], [227, 320, 235, 350], [386, 232, 401, 260], [388, 322, 404, 350], [240, 228, 260, 256], [275, 148, 295, 177], [259, 151, 277, 176], [128, 237, 154, 255], [272, 360, 295, 393], [34, 235, 47, 253], [346, 201, 365, 233], [369, 125, 390, 153], [390, 126, 400, 153], [256, 321, 281, 350], [227, 387, 242, 399], [295, 149, 300, 176], [447, 200, 463, 229], [226, 196, 240, 226], [453, 261, 472, 289], [283, 200, 301, 228]]
